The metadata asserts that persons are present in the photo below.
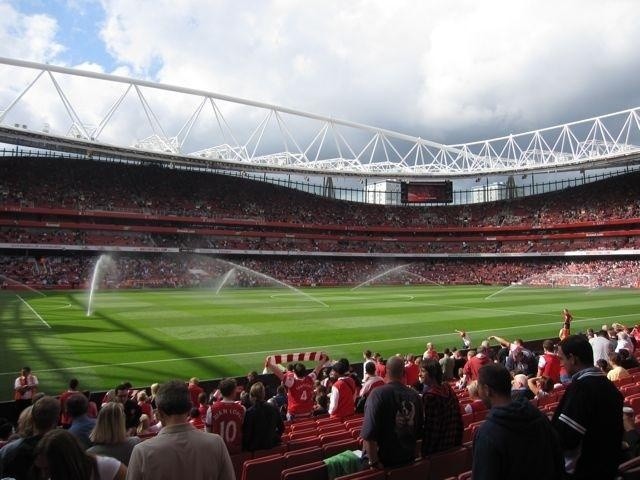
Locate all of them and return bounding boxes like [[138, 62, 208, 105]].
[[241, 381, 284, 451], [205, 377, 245, 456], [473, 362, 564, 479], [361, 354, 418, 469], [11, 366, 41, 401], [414, 359, 466, 455], [326, 363, 360, 420], [550, 334, 624, 478], [125, 379, 238, 479], [35, 428, 128, 479], [0, 156, 640, 290], [0, 306, 640, 480]]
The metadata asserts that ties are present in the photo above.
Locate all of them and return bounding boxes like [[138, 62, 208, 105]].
[[25, 377, 27, 385]]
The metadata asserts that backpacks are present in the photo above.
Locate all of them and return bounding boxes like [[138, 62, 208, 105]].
[[428, 386, 464, 451]]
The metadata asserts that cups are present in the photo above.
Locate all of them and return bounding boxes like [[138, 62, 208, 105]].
[[623, 407, 634, 421]]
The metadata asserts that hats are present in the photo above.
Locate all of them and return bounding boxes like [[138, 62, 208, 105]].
[[156, 381, 189, 407]]
